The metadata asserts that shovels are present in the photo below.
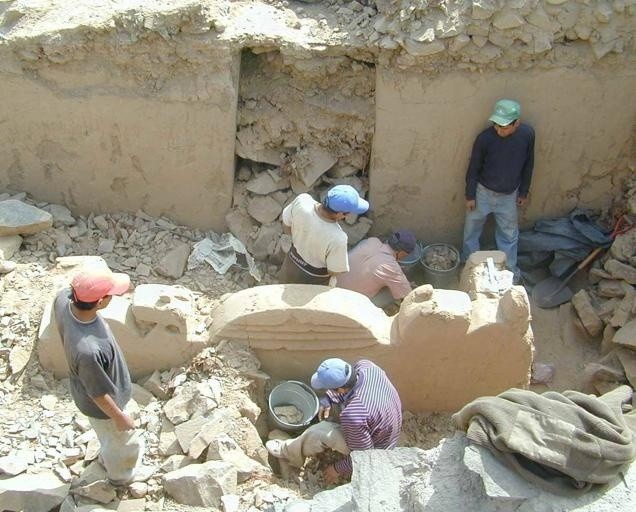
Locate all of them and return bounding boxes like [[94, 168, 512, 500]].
[[532, 213, 633, 308]]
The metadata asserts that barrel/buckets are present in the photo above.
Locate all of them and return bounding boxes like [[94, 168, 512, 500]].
[[268, 380, 320, 431], [388, 236, 421, 279], [419, 243, 461, 289]]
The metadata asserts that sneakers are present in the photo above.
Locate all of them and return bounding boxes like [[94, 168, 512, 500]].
[[514, 274, 533, 295], [110, 465, 153, 486], [265, 439, 288, 459]]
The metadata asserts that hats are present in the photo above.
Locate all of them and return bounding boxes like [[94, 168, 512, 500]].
[[310, 357, 351, 390], [322, 184, 369, 215], [392, 229, 416, 253], [488, 99, 521, 127], [70, 265, 130, 303]]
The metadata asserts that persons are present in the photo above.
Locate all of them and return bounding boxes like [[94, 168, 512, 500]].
[[264, 357, 402, 482], [461, 100, 536, 296], [277, 184, 371, 286], [333, 228, 415, 312], [53, 259, 155, 489]]
